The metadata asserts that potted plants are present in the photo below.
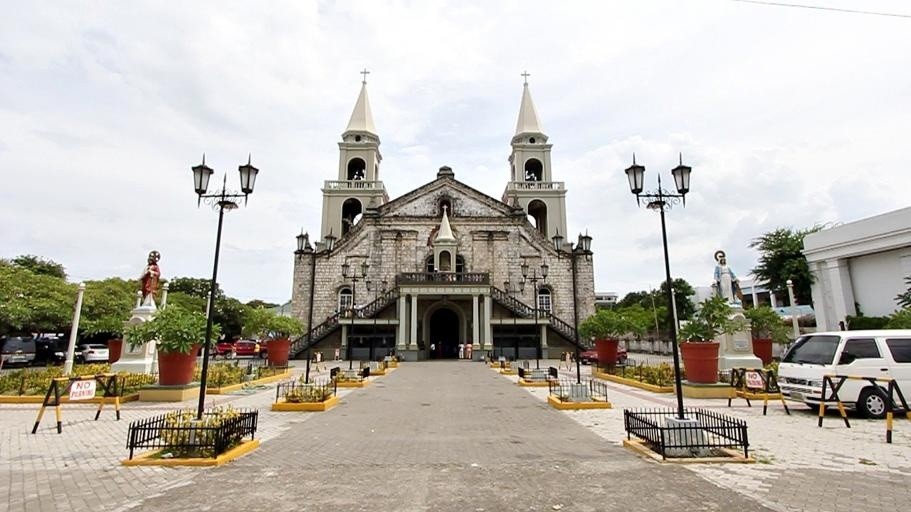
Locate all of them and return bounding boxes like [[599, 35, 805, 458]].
[[243, 309, 306, 369], [675, 294, 747, 384], [576, 307, 644, 370], [79, 314, 124, 365], [743, 305, 795, 369], [120, 303, 224, 386]]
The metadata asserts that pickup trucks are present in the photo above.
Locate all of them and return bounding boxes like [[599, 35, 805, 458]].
[[215, 340, 268, 359]]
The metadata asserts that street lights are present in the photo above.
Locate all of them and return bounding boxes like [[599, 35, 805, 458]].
[[624, 151, 691, 421], [292, 227, 336, 384], [550, 226, 592, 384], [520, 256, 548, 371], [190, 153, 258, 418], [339, 255, 369, 371], [503, 277, 524, 362], [366, 276, 388, 362]]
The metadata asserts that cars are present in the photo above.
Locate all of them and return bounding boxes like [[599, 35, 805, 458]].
[[77, 343, 109, 364], [580, 346, 627, 365], [0, 332, 36, 367], [31, 337, 84, 366]]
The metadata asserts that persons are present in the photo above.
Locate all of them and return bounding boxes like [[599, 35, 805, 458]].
[[457, 341, 465, 359], [430, 343, 436, 356], [253, 342, 260, 359], [465, 342, 472, 359], [141, 256, 161, 304], [713, 257, 741, 304], [231, 344, 237, 360]]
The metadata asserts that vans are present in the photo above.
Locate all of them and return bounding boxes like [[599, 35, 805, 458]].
[[776, 328, 911, 419]]
